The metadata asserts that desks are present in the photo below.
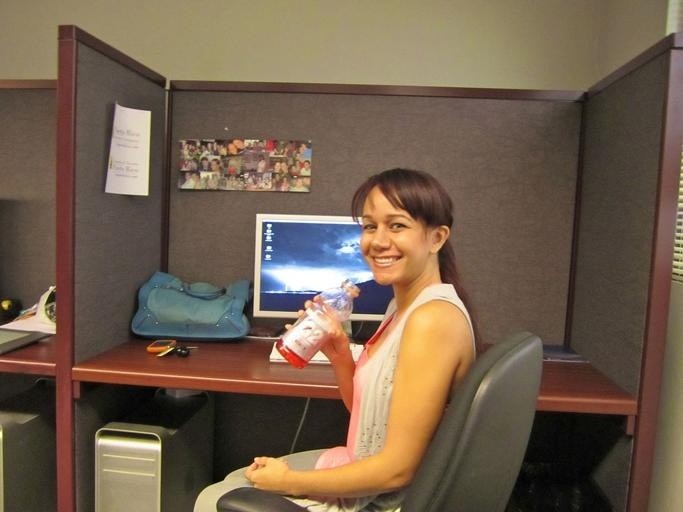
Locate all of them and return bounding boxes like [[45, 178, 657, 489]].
[[0, 334, 57, 377], [71, 325, 639, 417]]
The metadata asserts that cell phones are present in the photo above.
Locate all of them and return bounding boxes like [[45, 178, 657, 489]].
[[146, 338, 176, 353]]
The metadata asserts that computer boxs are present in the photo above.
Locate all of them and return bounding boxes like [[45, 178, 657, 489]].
[[94, 388, 216, 512]]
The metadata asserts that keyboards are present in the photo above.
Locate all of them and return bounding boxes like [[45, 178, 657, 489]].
[[269, 341, 365, 366]]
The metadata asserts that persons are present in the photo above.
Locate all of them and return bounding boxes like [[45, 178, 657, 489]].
[[180, 140, 311, 192], [193, 168, 475, 512]]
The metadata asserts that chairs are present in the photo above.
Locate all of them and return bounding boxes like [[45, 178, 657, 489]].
[[216, 329, 544, 512]]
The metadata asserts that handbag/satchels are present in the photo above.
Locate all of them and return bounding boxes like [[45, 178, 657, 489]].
[[131, 272, 250, 339]]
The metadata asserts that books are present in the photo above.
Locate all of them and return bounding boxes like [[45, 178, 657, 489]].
[[542, 344, 591, 365]]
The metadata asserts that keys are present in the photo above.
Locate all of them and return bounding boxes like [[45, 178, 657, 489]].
[[156, 344, 199, 358]]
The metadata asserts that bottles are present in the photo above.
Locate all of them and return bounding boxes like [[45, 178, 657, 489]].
[[275, 279, 361, 369]]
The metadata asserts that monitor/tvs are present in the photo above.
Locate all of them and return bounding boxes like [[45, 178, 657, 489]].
[[253, 213, 394, 322]]
